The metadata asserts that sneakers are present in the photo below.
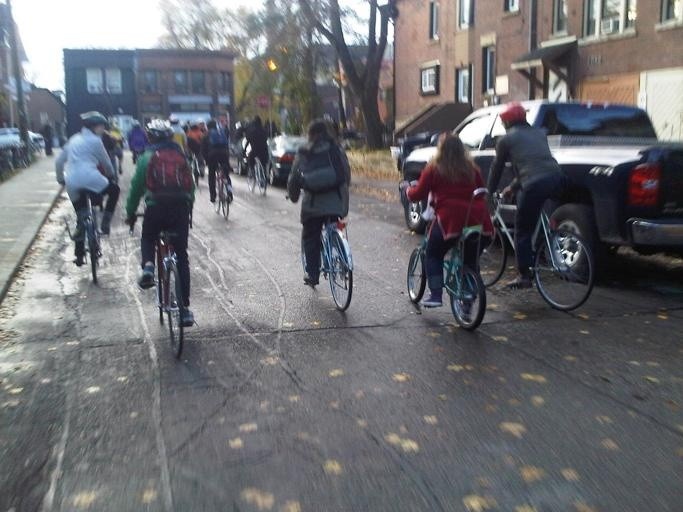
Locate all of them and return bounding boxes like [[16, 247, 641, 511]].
[[138, 266, 155, 287], [506, 275, 534, 290], [100, 221, 112, 234], [458, 302, 474, 321], [177, 311, 195, 326], [209, 191, 234, 202], [73, 255, 85, 266], [304, 272, 321, 284], [421, 295, 443, 307]]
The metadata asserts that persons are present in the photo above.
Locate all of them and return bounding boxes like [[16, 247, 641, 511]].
[[286, 119, 351, 284], [485, 102, 564, 287], [124, 118, 196, 326], [40, 120, 53, 154], [405, 130, 495, 306], [54, 109, 120, 265], [96, 117, 280, 203]]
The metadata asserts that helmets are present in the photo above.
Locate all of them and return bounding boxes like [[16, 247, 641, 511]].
[[80, 111, 108, 126], [170, 114, 227, 128], [131, 119, 141, 126], [145, 119, 174, 138]]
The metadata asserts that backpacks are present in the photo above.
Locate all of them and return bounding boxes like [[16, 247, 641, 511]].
[[297, 147, 345, 192], [146, 143, 193, 197]]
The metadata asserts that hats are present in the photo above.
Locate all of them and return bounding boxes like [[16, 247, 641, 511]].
[[499, 104, 527, 121]]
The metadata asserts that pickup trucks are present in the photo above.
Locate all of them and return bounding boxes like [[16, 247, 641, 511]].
[[402, 98, 682, 284]]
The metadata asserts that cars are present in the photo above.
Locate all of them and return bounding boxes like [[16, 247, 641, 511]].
[[228, 131, 309, 188], [1, 128, 45, 151]]
